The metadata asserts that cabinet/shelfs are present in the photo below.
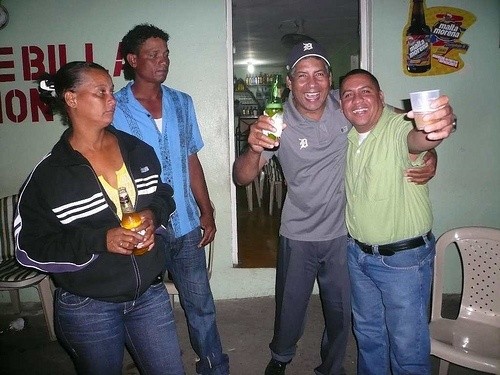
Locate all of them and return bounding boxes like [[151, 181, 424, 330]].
[[235, 84, 273, 105]]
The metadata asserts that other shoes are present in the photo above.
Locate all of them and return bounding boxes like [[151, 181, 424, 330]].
[[265, 358, 292, 375]]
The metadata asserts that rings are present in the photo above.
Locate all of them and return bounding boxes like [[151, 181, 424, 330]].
[[120, 241, 124, 247]]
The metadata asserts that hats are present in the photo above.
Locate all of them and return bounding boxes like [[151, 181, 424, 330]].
[[288, 41, 331, 68]]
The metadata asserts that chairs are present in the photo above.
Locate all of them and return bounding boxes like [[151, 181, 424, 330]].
[[428, 226, 500, 375], [163, 201, 216, 308], [0, 194, 57, 342], [236, 116, 258, 156]]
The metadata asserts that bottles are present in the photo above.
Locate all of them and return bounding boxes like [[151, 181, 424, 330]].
[[241, 72, 276, 116], [261, 74, 284, 152], [118, 187, 148, 256]]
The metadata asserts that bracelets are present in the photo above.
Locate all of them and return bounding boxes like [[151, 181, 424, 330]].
[[452, 118, 456, 130]]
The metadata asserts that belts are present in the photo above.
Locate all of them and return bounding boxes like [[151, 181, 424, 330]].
[[353, 229, 432, 256]]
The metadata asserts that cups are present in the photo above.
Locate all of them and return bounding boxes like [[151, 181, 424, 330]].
[[409, 88, 440, 131]]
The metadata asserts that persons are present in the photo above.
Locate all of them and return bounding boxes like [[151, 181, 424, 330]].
[[339, 67, 457, 375], [232, 41, 438, 375], [14, 61, 185, 375], [110, 23, 230, 375]]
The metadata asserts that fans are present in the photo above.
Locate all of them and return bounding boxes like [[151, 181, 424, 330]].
[[281, 20, 316, 48]]
